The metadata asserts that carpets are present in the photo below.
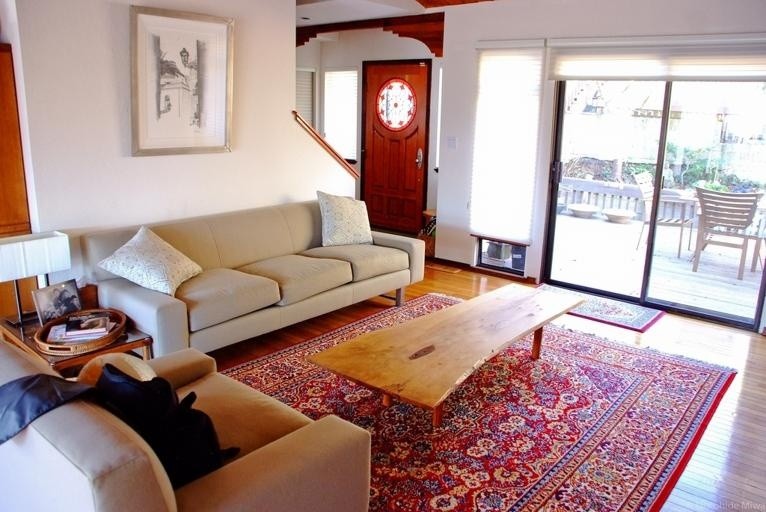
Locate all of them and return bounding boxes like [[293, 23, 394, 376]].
[[533, 282, 667, 334], [211, 291, 741, 512]]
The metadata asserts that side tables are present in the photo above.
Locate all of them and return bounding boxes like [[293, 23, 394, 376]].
[[0, 316, 152, 371]]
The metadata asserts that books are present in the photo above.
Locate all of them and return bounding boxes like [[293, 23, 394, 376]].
[[46, 311, 118, 345]]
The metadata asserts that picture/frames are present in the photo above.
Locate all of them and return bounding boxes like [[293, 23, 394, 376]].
[[29, 278, 84, 328], [128, 4, 235, 156]]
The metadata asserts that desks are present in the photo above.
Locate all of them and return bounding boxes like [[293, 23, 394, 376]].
[[694, 206, 765, 275]]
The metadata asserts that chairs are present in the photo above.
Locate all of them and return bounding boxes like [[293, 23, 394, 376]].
[[691, 186, 762, 280], [636, 170, 697, 261]]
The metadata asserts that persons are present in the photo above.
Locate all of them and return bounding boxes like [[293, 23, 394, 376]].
[[45, 289, 82, 318]]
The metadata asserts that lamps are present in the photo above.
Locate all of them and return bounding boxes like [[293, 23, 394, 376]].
[[0, 229, 71, 327]]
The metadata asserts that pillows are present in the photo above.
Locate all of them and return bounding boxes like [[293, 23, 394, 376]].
[[315, 191, 373, 248], [95, 223, 203, 298]]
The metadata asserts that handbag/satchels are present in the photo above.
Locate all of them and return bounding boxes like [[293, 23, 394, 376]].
[[89, 363, 241, 491]]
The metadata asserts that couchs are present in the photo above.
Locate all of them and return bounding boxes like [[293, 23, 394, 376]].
[[80, 190, 427, 366], [1, 337, 372, 512]]
